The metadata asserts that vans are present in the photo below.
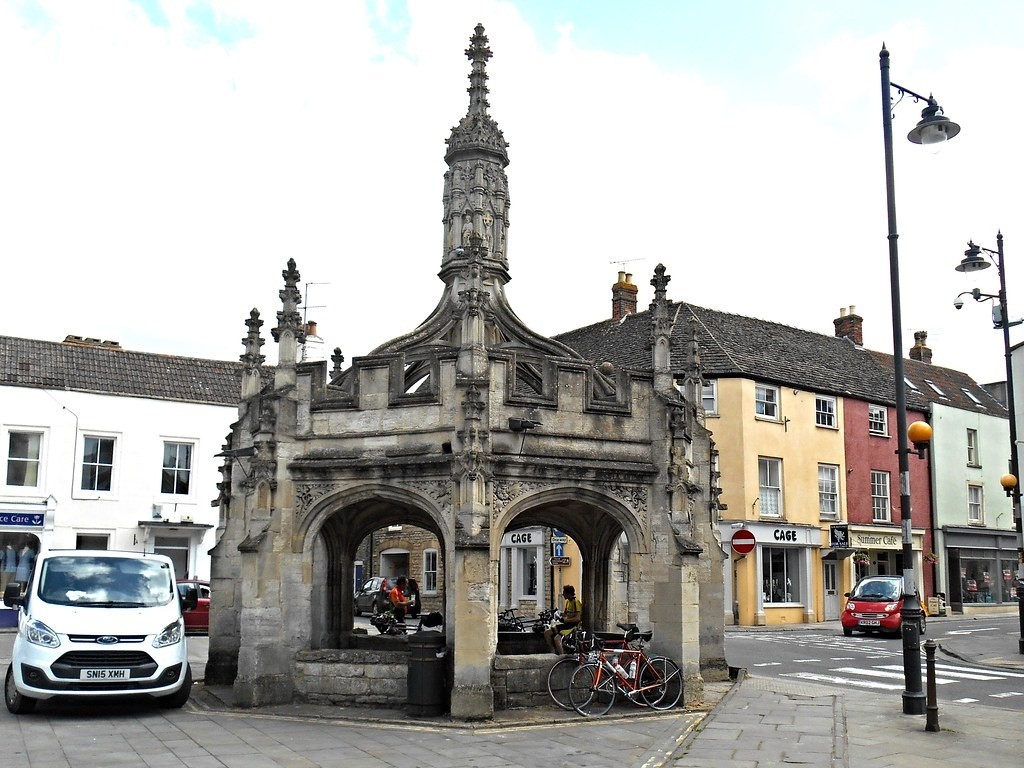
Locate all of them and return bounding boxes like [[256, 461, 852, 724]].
[[4, 548, 198, 715]]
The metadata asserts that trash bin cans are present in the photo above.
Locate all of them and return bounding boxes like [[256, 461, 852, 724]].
[[404, 630, 451, 718]]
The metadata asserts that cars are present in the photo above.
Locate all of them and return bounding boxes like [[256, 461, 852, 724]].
[[352, 576, 414, 622], [840, 575, 926, 638], [175, 579, 212, 636]]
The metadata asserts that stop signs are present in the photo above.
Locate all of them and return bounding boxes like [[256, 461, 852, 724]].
[[731, 529, 756, 554]]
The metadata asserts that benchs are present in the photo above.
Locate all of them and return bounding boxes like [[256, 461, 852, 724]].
[[594, 630, 653, 642], [498, 631, 545, 640]]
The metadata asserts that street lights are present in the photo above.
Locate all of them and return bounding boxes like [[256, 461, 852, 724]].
[[876, 41, 962, 717], [954, 226, 1024, 655]]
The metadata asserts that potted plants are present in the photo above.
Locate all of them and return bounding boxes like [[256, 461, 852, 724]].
[[370, 610, 399, 633]]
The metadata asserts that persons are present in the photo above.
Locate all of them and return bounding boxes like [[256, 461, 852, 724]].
[[0, 543, 20, 597], [388, 571, 414, 635], [200, 586, 213, 600], [15, 544, 36, 582], [544, 584, 584, 656]]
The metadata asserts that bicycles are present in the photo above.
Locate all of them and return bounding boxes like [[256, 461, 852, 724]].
[[499, 607, 561, 635], [567, 630, 682, 718], [548, 623, 667, 711], [370, 613, 406, 636]]
